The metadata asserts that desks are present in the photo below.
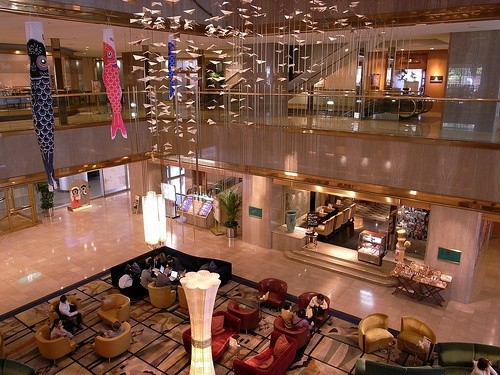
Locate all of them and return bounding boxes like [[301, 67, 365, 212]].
[[389, 266, 449, 307], [305, 202, 344, 225]]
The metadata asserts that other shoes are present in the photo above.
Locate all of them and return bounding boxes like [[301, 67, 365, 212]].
[[76, 324, 83, 330]]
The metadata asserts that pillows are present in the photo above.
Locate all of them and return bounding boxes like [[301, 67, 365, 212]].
[[210, 315, 225, 335], [259, 355, 274, 370], [273, 334, 289, 357]]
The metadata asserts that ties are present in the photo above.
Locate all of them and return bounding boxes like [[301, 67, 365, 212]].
[[155, 260, 156, 266]]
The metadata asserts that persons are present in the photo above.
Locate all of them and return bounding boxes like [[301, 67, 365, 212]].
[[49, 318, 78, 348], [155, 268, 178, 301], [470, 358, 490, 375], [292, 309, 314, 331], [166, 250, 185, 272], [141, 263, 154, 289], [92, 322, 125, 348], [306, 294, 328, 325], [281, 303, 294, 329], [59, 295, 83, 335]]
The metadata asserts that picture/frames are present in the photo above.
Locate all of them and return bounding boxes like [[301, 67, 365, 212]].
[[437, 247, 462, 265]]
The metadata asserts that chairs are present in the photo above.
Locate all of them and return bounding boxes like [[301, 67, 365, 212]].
[[358, 313, 394, 358], [315, 202, 356, 239], [396, 316, 437, 366]]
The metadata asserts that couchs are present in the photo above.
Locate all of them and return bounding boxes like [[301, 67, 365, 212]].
[[227, 299, 263, 334], [96, 294, 131, 329], [297, 292, 330, 323], [355, 341, 500, 375], [34, 324, 76, 363], [274, 315, 313, 351], [48, 295, 85, 326], [255, 278, 288, 310], [182, 311, 242, 368], [91, 322, 132, 364], [0, 358, 36, 375], [110, 245, 232, 305], [233, 330, 298, 374], [177, 285, 188, 308], [147, 281, 176, 308]]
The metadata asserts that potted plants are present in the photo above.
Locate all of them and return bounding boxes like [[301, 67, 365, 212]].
[[32, 180, 54, 218], [215, 186, 243, 238]]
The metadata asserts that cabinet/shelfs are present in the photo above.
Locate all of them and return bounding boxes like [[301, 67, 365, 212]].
[[357, 229, 388, 266]]
